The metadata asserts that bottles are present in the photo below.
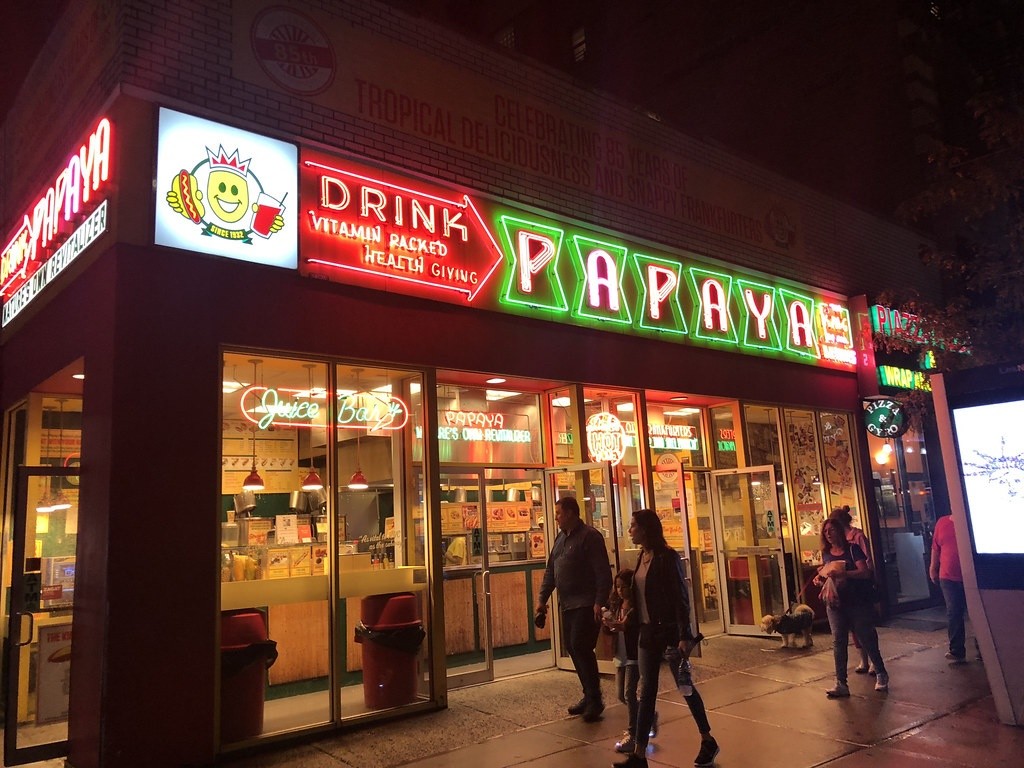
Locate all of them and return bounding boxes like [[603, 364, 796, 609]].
[[678, 658, 692, 696], [601, 607, 618, 631]]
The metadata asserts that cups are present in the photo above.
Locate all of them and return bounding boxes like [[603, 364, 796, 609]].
[[831, 560, 845, 571], [227, 511, 235, 522]]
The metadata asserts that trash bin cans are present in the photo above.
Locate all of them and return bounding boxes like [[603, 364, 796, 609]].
[[220, 609, 272, 742], [356, 594, 425, 709], [729, 558, 770, 626]]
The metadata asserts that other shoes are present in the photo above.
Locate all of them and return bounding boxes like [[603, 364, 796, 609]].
[[694, 736, 720, 766], [945, 653, 966, 660], [868, 662, 876, 673], [648, 711, 658, 737], [976, 654, 981, 660], [611, 752, 649, 768], [855, 663, 871, 673], [615, 737, 636, 753], [874, 672, 889, 689], [826, 685, 850, 696], [568, 694, 606, 721]]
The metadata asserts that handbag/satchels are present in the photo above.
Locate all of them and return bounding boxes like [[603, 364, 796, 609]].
[[850, 544, 880, 599]]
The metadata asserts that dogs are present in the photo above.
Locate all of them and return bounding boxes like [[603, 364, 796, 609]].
[[758, 604, 816, 647]]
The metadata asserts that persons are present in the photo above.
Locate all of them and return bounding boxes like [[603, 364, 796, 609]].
[[602, 569, 658, 752], [441, 535, 468, 566], [534, 497, 613, 721], [929, 515, 982, 660], [610, 509, 720, 768], [812, 506, 888, 697]]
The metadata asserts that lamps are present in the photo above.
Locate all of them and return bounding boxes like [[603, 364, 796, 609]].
[[50, 399, 72, 509], [302, 362, 324, 490], [242, 360, 265, 490], [348, 369, 369, 489], [36, 405, 55, 512]]
